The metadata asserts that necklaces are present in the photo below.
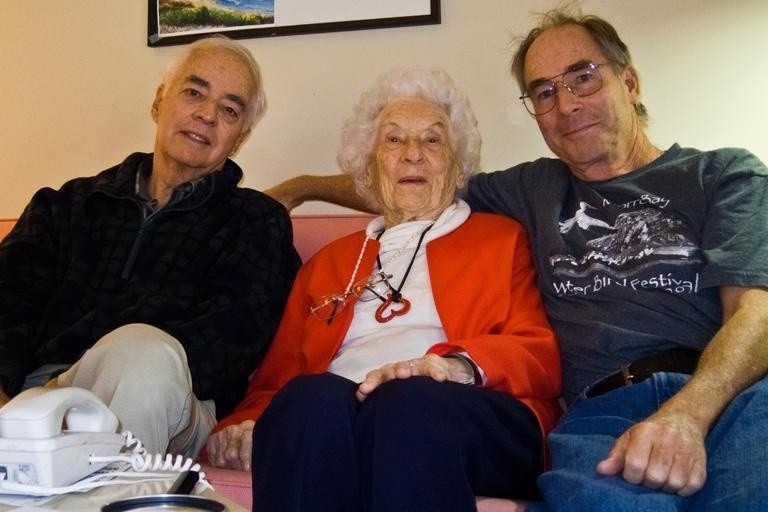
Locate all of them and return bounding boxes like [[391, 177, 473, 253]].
[[368, 219, 435, 326]]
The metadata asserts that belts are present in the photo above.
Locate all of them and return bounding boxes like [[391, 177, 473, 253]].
[[583, 350, 680, 400]]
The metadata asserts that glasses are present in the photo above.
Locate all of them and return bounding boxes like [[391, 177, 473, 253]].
[[518, 61, 613, 117], [310, 271, 393, 325]]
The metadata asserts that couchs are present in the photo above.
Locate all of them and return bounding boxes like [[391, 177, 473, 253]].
[[0, 213, 524, 512]]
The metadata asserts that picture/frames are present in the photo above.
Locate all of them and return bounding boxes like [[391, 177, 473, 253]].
[[148, 0, 442, 47]]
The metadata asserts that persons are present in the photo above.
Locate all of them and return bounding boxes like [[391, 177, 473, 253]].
[[0, 31, 302, 472], [256, 1, 768, 511], [199, 63, 568, 511]]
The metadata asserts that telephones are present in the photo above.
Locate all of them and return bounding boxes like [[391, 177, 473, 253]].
[[0, 386, 126, 488]]
